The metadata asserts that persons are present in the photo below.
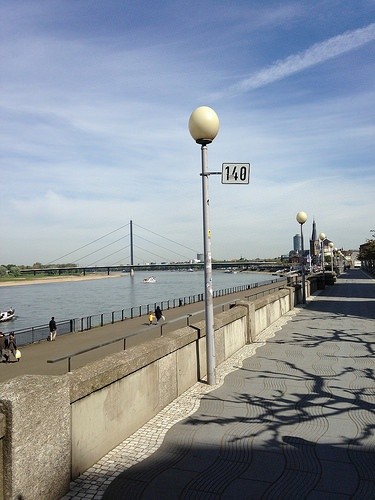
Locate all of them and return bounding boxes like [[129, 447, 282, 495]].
[[49, 317, 57, 341], [149, 313, 155, 325], [0, 332, 20, 364], [155, 306, 162, 323]]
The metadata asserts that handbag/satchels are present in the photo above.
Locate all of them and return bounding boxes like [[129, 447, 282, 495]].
[[161, 315, 165, 320], [15, 349, 21, 358]]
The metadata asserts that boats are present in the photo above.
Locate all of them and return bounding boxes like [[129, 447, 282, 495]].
[[0, 307, 16, 323], [141, 276, 157, 284]]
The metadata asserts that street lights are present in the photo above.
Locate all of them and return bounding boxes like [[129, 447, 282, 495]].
[[334, 248, 338, 277], [188, 105, 221, 386], [296, 211, 309, 304], [319, 233, 326, 290], [329, 243, 334, 271]]
